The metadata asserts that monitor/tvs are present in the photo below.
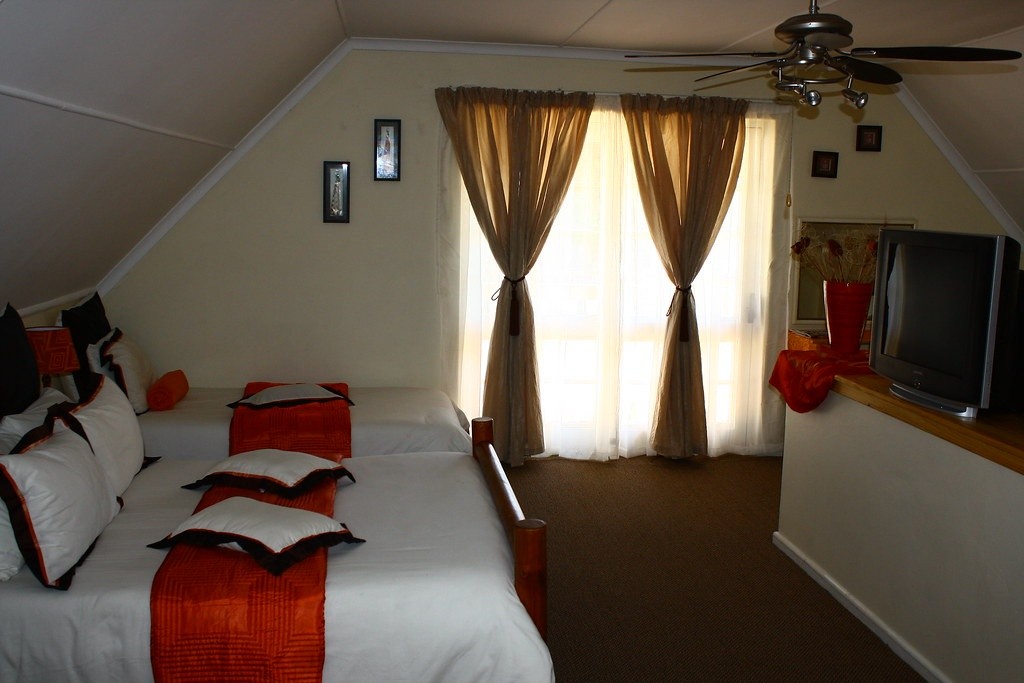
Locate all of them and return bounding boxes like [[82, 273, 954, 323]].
[[868, 228, 1024, 419]]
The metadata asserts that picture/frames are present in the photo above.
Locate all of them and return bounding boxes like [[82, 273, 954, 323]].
[[323, 160, 351, 223], [855, 125, 882, 153], [811, 151, 839, 178], [374, 118, 402, 181], [791, 216, 919, 326]]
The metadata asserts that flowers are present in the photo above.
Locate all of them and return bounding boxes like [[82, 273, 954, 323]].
[[791, 235, 881, 283]]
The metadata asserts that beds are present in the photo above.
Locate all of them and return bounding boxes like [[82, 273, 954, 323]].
[[0, 304, 555, 683], [55, 291, 473, 459]]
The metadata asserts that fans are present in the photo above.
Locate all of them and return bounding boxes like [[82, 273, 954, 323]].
[[623, 0, 1022, 85]]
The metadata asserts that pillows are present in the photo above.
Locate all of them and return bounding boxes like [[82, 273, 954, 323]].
[[180, 449, 357, 502], [145, 496, 366, 578], [0, 304, 40, 416], [46, 370, 162, 495], [99, 327, 151, 416], [8, 388, 74, 438], [147, 369, 189, 411], [62, 292, 111, 370], [0, 423, 23, 583], [224, 382, 356, 413], [87, 329, 115, 384], [0, 411, 125, 592]]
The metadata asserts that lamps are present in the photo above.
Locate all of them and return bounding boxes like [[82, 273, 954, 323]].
[[774, 77, 868, 109]]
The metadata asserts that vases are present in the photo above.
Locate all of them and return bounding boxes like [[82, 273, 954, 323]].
[[821, 279, 875, 355]]
